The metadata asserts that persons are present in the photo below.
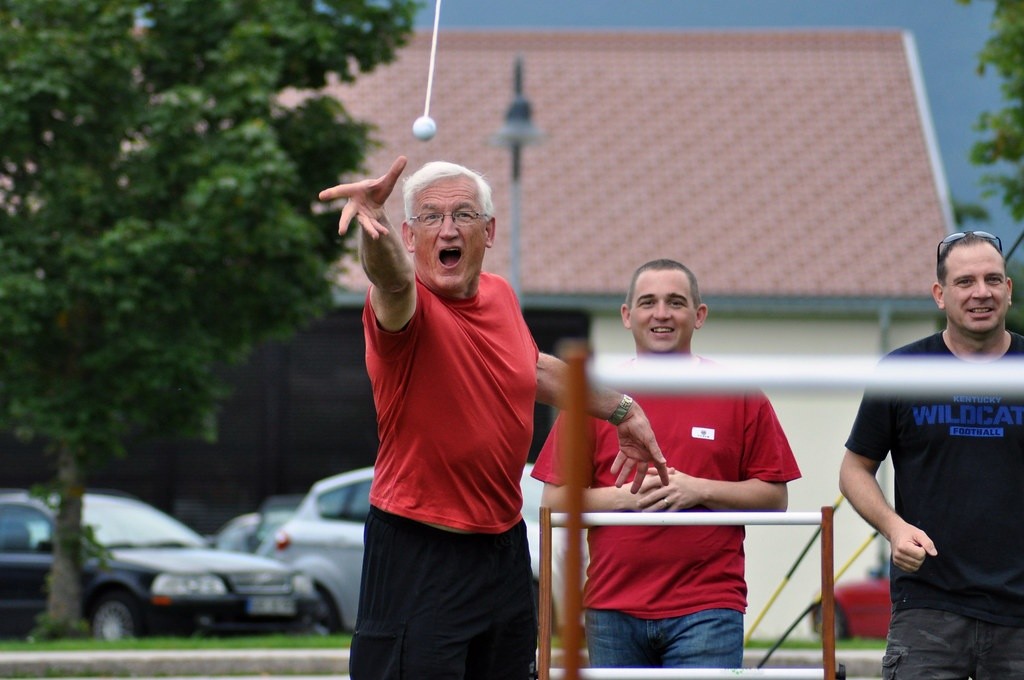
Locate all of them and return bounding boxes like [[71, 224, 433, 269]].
[[319, 156, 670, 680], [839, 231, 1024, 680], [530, 259, 802, 669]]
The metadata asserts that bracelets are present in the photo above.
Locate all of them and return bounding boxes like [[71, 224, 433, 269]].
[[608, 394, 633, 426]]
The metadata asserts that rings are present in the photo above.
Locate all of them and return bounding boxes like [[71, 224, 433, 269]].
[[663, 499, 670, 506]]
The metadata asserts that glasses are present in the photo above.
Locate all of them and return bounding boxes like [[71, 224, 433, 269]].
[[936, 230, 1003, 269], [410, 210, 488, 228]]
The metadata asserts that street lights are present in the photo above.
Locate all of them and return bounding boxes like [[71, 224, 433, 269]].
[[485, 53, 547, 291]]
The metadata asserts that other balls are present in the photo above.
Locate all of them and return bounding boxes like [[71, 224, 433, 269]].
[[412, 116, 437, 141]]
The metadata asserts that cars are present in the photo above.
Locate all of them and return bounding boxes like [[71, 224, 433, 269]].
[[0, 486, 296, 644], [249, 456, 658, 638], [809, 561, 919, 640]]
